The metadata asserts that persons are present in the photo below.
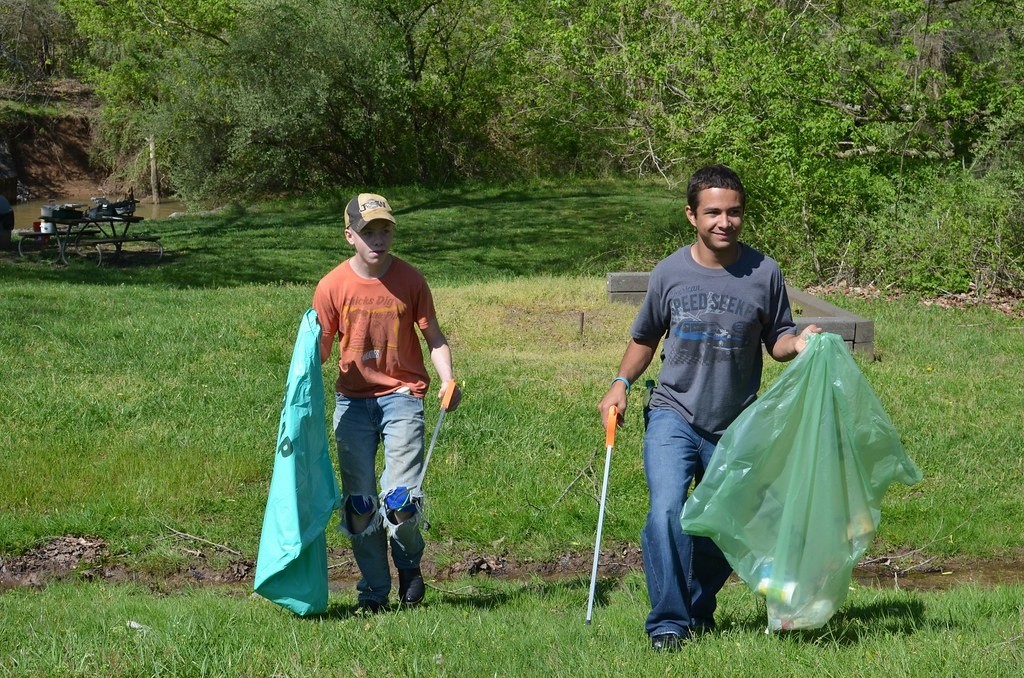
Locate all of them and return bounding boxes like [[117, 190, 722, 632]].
[[312, 193, 462, 619], [597, 165, 824, 652]]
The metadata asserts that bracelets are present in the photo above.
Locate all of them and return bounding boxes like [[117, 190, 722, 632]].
[[610, 376, 631, 396]]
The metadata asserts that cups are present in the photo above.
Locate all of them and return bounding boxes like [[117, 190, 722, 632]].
[[759, 577, 800, 609]]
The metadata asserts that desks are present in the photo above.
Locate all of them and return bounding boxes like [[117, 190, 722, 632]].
[[38, 214, 144, 267]]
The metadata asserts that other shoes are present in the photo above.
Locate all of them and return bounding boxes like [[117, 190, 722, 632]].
[[693, 625, 713, 635], [652, 633, 686, 654]]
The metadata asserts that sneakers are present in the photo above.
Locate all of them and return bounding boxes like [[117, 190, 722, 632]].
[[398, 567, 425, 604], [349, 599, 382, 617]]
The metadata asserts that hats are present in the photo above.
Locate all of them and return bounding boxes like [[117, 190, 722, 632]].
[[344, 193, 397, 233]]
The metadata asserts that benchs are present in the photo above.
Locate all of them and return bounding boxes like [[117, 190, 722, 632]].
[[18, 230, 163, 267]]
